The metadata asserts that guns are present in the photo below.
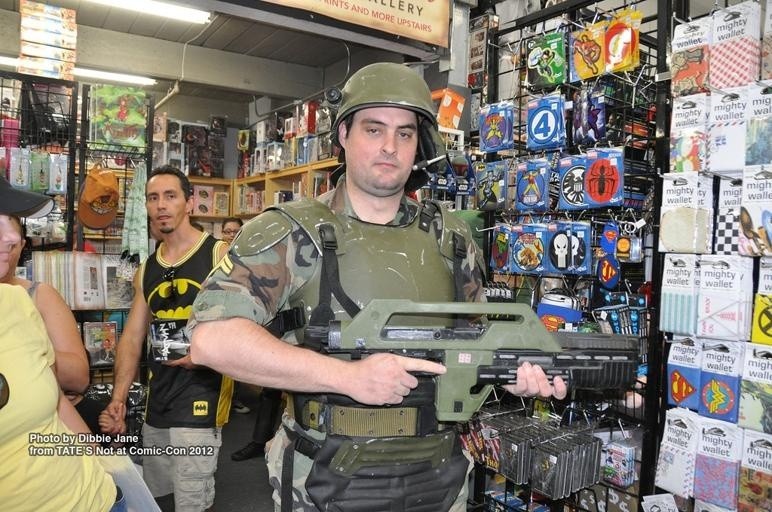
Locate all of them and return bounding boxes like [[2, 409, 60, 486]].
[[293, 299, 642, 422]]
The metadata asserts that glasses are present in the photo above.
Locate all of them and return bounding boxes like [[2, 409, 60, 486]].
[[223, 230, 239, 235]]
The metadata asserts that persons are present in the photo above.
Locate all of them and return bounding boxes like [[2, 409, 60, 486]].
[[2, 216, 91, 394], [189, 64, 569, 510], [101, 164, 232, 511], [220, 219, 250, 415], [64, 347, 136, 455], [231, 387, 282, 460], [0, 175, 130, 512], [263, 299, 644, 437]]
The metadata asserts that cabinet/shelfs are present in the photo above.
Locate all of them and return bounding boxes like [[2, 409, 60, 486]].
[[54, 158, 342, 240]]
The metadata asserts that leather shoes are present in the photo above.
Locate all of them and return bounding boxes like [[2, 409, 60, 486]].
[[232, 441, 264, 460]]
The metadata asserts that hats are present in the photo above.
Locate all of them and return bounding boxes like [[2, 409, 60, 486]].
[[78, 162, 119, 229], [0, 176, 54, 218]]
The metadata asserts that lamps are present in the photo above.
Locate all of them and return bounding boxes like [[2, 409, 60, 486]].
[[91, 0, 215, 25]]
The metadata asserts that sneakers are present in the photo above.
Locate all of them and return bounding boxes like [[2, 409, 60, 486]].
[[231, 404, 250, 413]]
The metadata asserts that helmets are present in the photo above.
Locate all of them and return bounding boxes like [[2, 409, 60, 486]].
[[331, 63, 437, 146]]
